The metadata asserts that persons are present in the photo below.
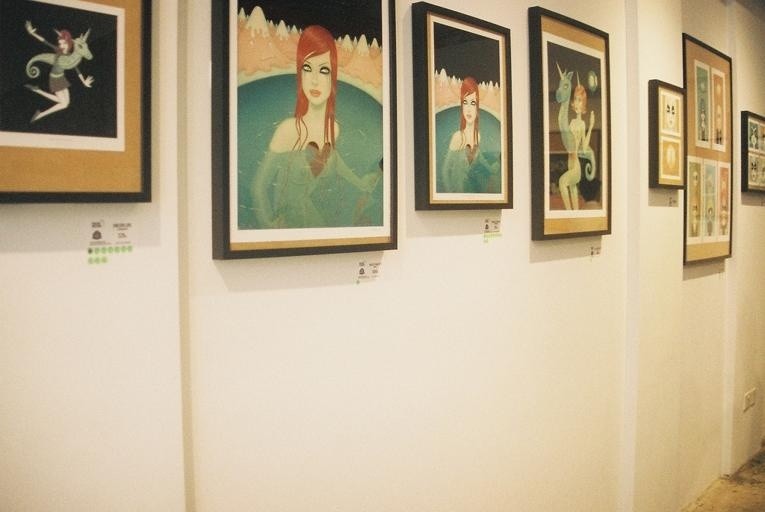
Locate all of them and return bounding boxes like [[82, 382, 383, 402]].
[[559, 84, 594, 210], [24, 20, 95, 123], [251, 26, 375, 229], [440, 76, 499, 193]]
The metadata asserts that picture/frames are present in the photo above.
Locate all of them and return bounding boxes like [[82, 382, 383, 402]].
[[408, 1, 516, 212], [646, 76, 688, 191], [740, 107, 765, 196], [526, 3, 613, 243], [682, 30, 736, 265], [0, 1, 155, 207], [207, 0, 401, 262]]
